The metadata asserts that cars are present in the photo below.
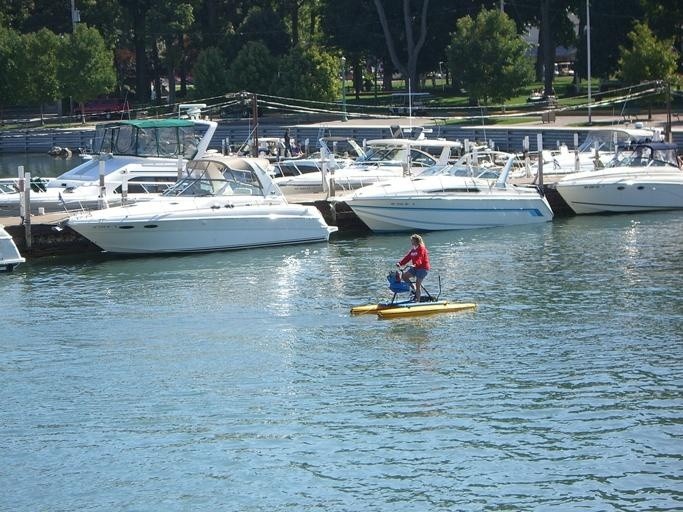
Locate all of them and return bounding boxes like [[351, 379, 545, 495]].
[[554, 61, 575, 76], [528, 86, 556, 101]]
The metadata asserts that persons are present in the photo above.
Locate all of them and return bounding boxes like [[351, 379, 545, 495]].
[[397, 234, 429, 304], [285, 127, 293, 158], [304, 136, 310, 158]]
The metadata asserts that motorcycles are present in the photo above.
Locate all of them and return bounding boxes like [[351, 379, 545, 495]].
[[350, 263, 476, 318]]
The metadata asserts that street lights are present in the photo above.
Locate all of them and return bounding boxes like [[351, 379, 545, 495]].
[[340, 56, 346, 111]]
